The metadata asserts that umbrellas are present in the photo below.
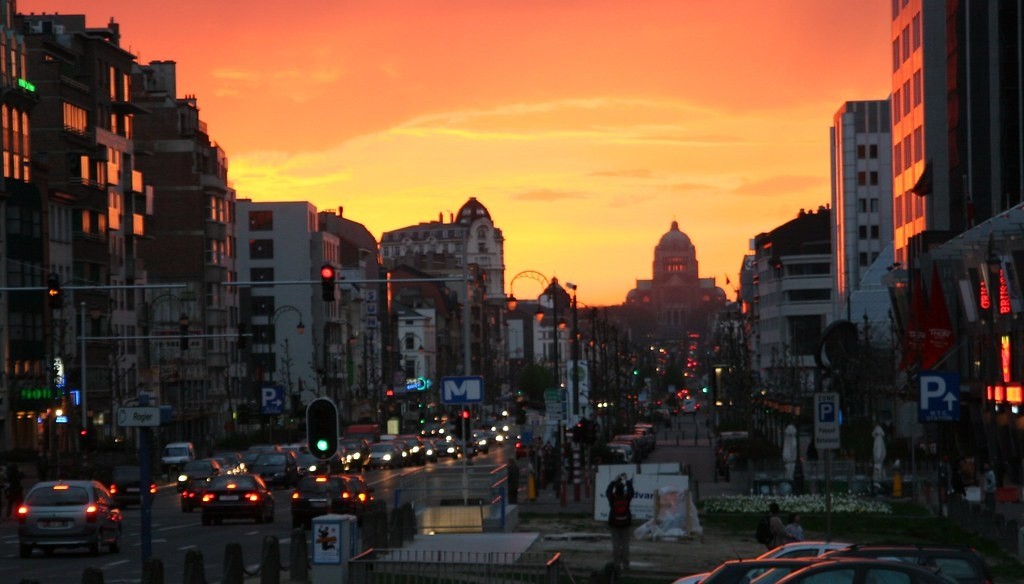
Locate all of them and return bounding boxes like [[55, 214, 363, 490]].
[[781, 425, 798, 480], [872, 426, 886, 483]]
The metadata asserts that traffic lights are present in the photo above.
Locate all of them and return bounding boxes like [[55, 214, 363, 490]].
[[48, 272, 64, 308], [306, 398, 340, 462], [320, 264, 342, 302], [456, 406, 471, 441]]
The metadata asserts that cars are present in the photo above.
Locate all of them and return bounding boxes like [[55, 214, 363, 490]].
[[111, 464, 158, 508], [18, 480, 123, 559], [176, 423, 509, 512], [200, 474, 274, 526], [671, 541, 992, 584], [290, 474, 374, 530]]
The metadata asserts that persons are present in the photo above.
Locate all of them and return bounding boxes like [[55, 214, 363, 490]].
[[939, 455, 951, 496], [766, 503, 796, 551], [983, 462, 996, 511], [950, 466, 967, 499], [807, 438, 818, 461], [784, 512, 804, 542], [0, 463, 24, 517], [37, 453, 48, 482], [992, 455, 1007, 486], [909, 443, 926, 471], [527, 442, 553, 474], [605, 473, 634, 569]]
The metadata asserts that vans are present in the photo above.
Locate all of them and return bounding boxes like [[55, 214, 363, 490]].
[[161, 441, 196, 473]]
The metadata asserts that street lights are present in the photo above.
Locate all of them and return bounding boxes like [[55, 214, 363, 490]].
[[398, 331, 424, 432], [536, 291, 643, 484], [329, 320, 357, 405], [507, 270, 567, 499], [267, 305, 306, 447], [366, 328, 394, 424]]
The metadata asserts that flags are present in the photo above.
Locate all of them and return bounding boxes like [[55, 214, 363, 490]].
[[921, 261, 956, 370], [899, 270, 927, 372]]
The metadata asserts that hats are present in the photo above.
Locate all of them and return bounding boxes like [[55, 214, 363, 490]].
[[615, 483, 624, 490]]
